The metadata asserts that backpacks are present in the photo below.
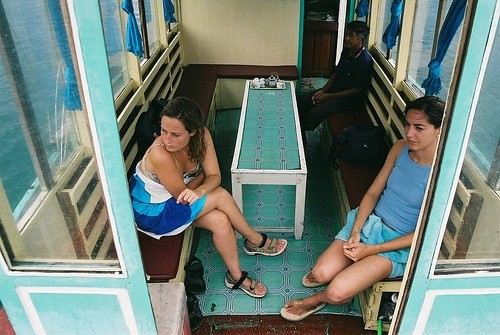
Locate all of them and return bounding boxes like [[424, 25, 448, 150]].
[[135, 98, 168, 155]]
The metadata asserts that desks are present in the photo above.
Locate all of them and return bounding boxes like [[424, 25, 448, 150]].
[[230, 79, 309, 240]]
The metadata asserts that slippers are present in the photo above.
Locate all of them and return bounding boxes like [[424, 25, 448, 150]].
[[302, 273, 324, 287], [280, 298, 326, 321]]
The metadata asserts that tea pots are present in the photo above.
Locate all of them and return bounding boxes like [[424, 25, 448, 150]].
[[266, 75, 279, 88]]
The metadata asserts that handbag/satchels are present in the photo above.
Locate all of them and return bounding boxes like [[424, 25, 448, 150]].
[[329, 125, 386, 170]]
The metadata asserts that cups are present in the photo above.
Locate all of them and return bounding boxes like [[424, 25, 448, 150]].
[[277, 82, 284, 88], [252, 78, 265, 88]]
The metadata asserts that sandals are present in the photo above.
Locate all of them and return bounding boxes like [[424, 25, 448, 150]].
[[225, 271, 267, 298], [244, 233, 288, 257]]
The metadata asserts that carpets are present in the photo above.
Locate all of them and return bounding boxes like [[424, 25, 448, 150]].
[[191, 105, 363, 319]]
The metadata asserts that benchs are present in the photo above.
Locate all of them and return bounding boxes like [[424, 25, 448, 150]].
[[326, 45, 484, 332], [105, 64, 299, 279]]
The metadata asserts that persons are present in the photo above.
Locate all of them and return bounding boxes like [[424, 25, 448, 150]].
[[129, 97, 287, 298], [297, 21, 373, 131], [280, 96, 446, 321]]
